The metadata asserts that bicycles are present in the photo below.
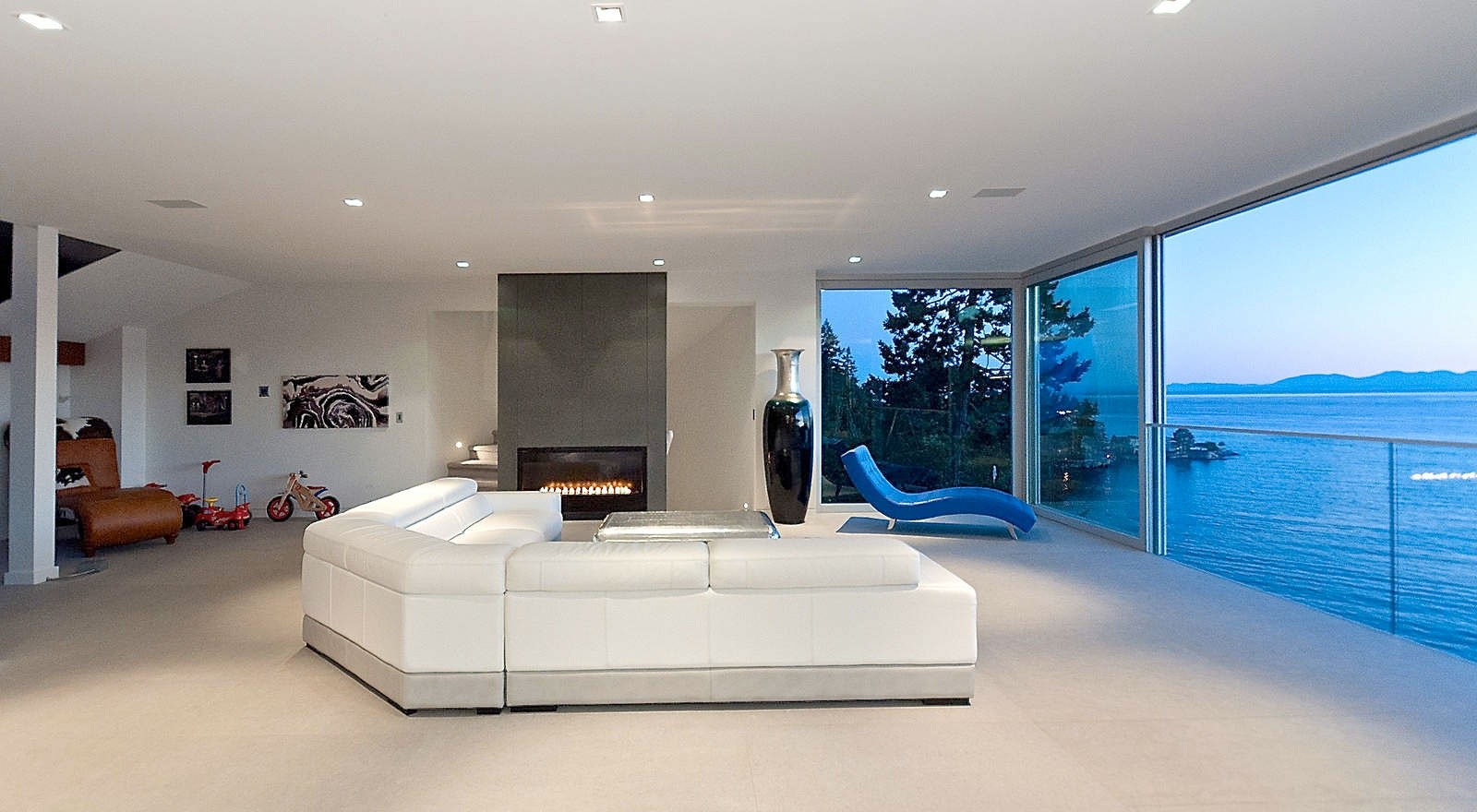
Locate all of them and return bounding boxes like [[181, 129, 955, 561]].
[[265, 470, 341, 520]]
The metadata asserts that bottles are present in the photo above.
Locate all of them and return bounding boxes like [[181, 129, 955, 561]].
[[762, 347, 814, 525]]
